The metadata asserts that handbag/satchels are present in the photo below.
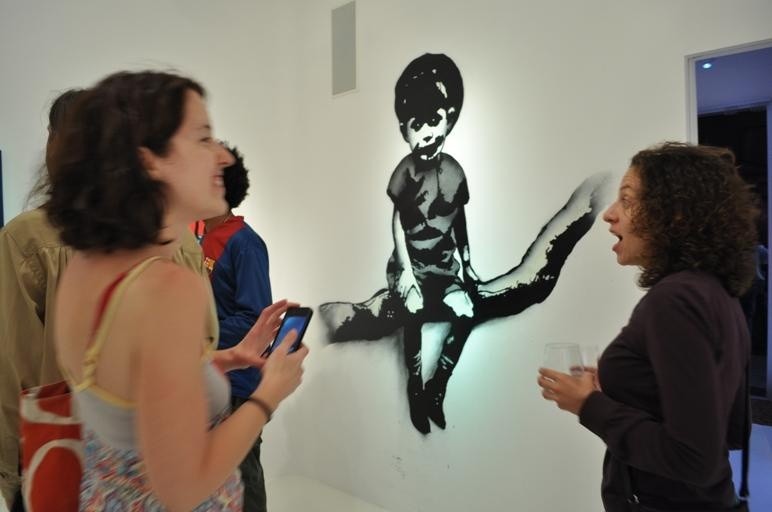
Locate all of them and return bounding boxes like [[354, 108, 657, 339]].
[[15, 341, 83, 512]]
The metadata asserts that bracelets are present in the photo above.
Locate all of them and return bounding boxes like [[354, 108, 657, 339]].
[[241, 395, 273, 425]]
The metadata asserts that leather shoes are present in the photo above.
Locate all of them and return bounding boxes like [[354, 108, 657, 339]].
[[408, 374, 453, 437]]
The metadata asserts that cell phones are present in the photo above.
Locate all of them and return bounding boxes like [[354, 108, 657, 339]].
[[267, 306, 312, 355]]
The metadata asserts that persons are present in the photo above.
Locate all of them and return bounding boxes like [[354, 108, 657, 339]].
[[1, 89, 98, 512], [197, 137, 274, 512], [378, 52, 487, 440], [535, 140, 758, 511], [39, 68, 310, 512]]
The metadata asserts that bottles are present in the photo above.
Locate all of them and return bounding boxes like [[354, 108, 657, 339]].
[[540, 342, 585, 396]]
